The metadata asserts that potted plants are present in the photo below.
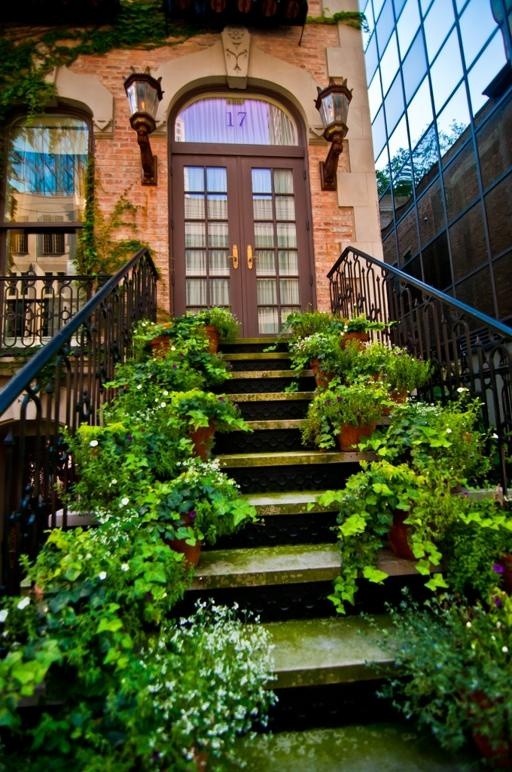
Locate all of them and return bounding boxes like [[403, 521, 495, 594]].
[[1, 582, 281, 772], [54, 307, 267, 615], [266, 307, 512, 616], [353, 491, 510, 769]]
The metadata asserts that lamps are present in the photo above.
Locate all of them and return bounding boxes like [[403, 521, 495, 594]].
[[314, 76, 354, 191], [121, 65, 166, 185]]
